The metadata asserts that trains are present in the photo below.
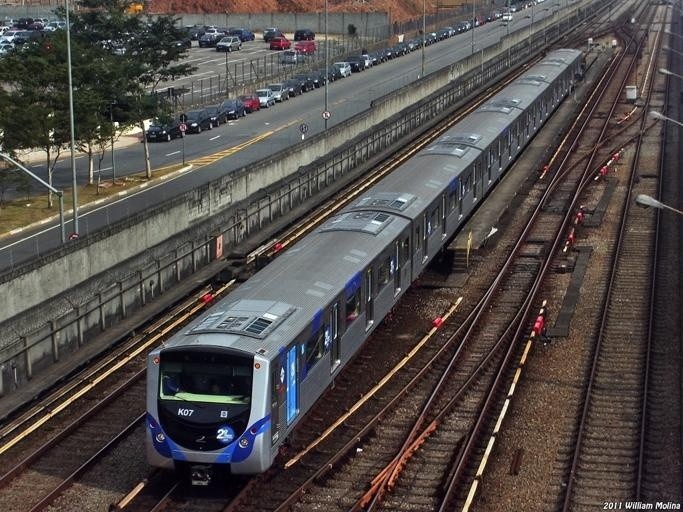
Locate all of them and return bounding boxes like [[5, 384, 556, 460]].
[[142, 46, 592, 491]]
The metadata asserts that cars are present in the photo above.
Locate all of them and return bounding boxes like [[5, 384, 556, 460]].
[[0, 16, 66, 57]]
